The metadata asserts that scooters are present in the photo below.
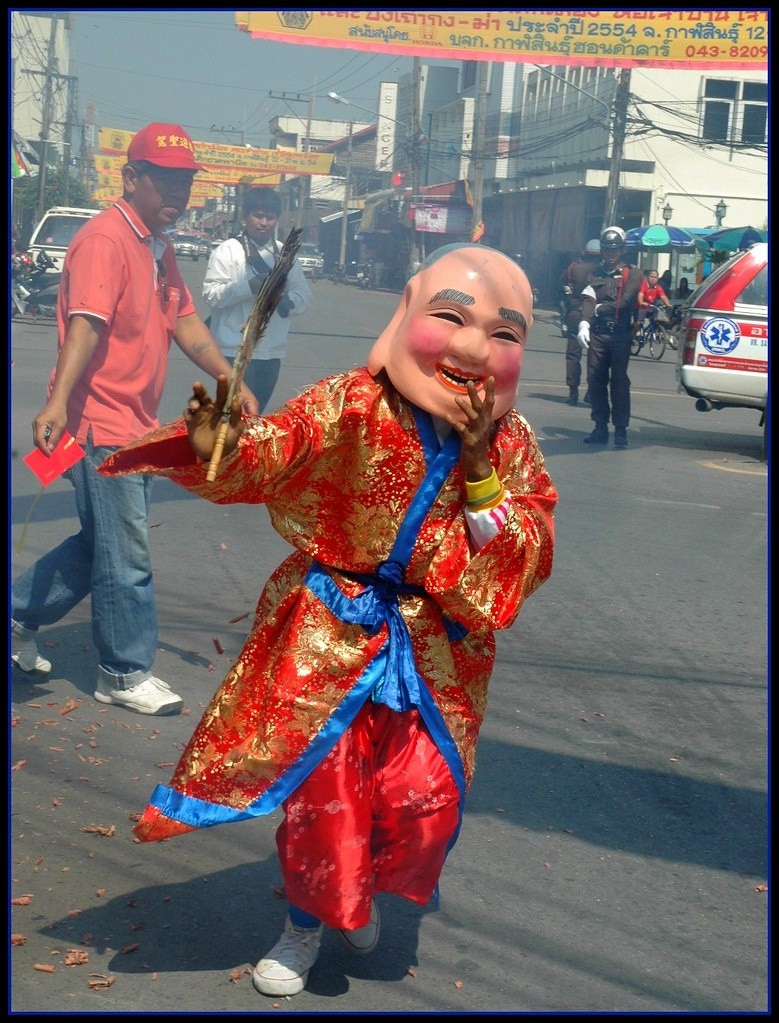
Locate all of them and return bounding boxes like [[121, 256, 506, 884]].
[[332, 261, 349, 286], [351, 259, 375, 291], [12, 244, 63, 318]]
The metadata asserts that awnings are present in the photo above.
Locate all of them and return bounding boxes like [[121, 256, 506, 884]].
[[12, 137, 58, 179], [357, 181, 474, 235]]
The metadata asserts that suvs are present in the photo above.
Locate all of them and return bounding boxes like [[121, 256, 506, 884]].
[[25, 202, 102, 275], [296, 243, 325, 279], [677, 242, 769, 414], [171, 231, 221, 262]]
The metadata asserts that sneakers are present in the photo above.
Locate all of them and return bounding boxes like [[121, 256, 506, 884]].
[[12, 620, 52, 678], [335, 895, 381, 955], [94, 664, 184, 716], [254, 915, 323, 995]]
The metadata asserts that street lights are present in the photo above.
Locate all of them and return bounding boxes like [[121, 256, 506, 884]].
[[660, 203, 674, 228], [713, 199, 730, 227], [326, 91, 418, 281]]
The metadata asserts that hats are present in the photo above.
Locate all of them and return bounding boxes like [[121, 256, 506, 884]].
[[585, 238, 602, 255], [129, 122, 209, 173], [600, 226, 627, 248]]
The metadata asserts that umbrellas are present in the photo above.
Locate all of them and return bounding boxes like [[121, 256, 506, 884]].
[[621, 223, 768, 261]]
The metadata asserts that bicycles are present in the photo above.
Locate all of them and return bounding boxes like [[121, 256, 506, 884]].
[[630, 301, 681, 361], [311, 264, 328, 283]]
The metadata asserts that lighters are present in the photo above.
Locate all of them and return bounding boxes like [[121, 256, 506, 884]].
[[45, 428, 50, 436]]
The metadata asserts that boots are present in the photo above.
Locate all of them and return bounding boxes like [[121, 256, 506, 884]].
[[584, 420, 609, 443], [566, 386, 579, 404], [614, 427, 628, 447], [583, 392, 592, 403]]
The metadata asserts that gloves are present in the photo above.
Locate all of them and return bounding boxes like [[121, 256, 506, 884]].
[[576, 321, 590, 349]]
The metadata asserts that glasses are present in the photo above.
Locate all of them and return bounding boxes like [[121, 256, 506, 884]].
[[155, 258, 171, 303]]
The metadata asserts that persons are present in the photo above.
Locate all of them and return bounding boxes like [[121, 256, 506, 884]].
[[9, 123, 260, 717], [202, 188, 312, 418], [576, 226, 642, 447], [98, 242, 556, 996], [659, 270, 695, 330], [375, 258, 384, 288], [559, 239, 604, 405], [631, 270, 672, 346]]
